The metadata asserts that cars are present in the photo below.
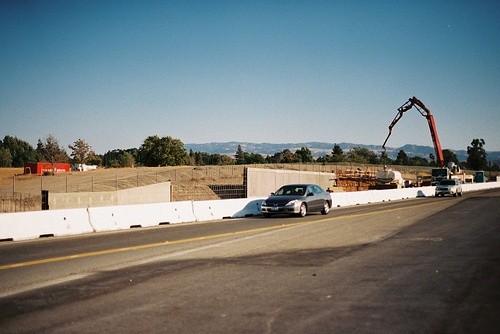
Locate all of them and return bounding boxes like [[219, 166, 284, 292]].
[[262, 183, 332, 217], [435, 178, 463, 198]]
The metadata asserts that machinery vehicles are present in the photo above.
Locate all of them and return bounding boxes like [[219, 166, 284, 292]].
[[380, 97, 474, 186]]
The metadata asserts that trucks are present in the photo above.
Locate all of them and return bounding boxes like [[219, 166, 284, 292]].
[[373, 170, 405, 190]]
